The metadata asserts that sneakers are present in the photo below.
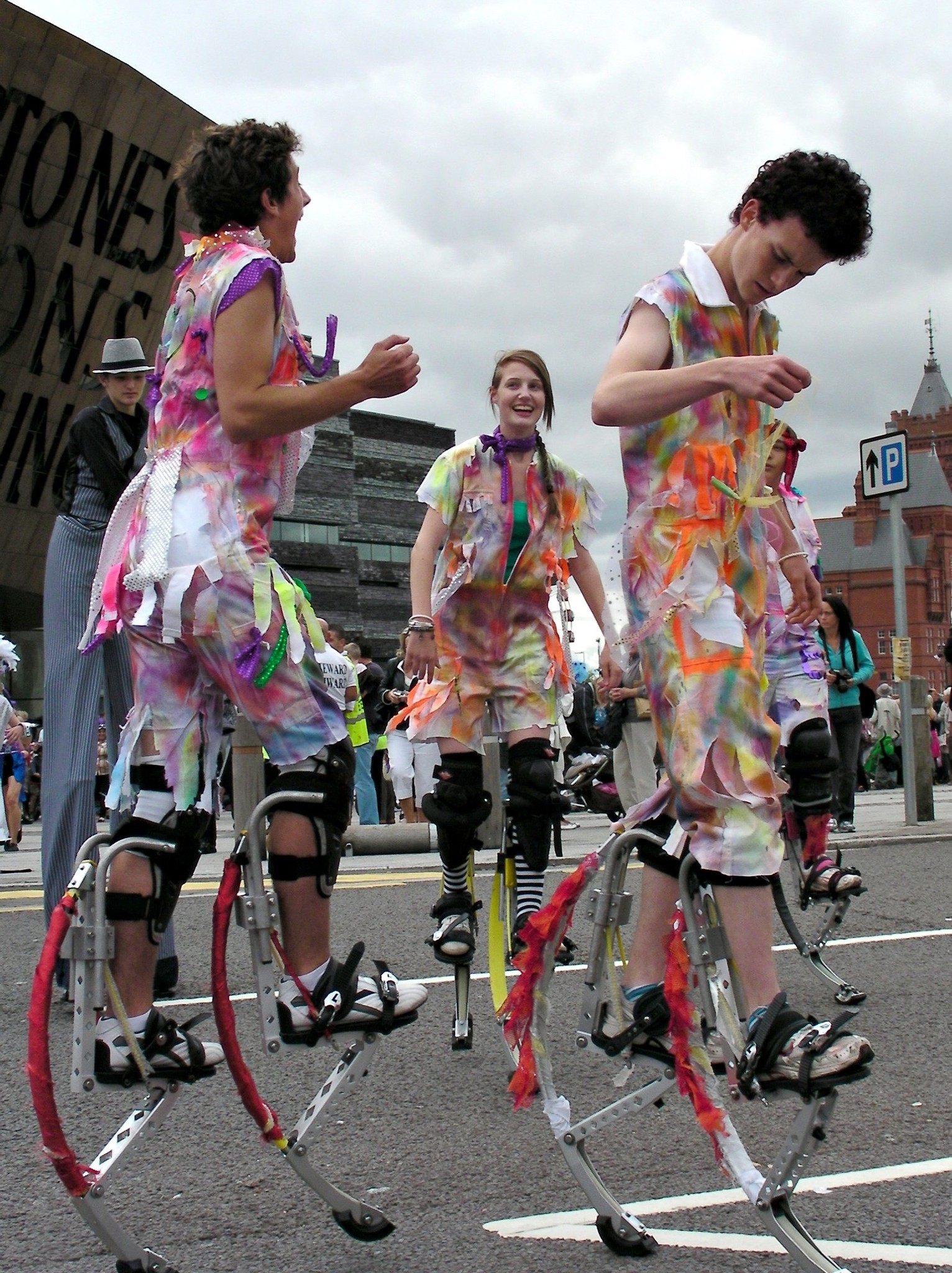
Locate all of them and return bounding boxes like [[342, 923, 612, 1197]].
[[433, 914, 474, 955], [607, 984, 728, 1065], [743, 1019, 874, 1080], [504, 914, 575, 968], [801, 852, 864, 891], [276, 942, 427, 1043], [93, 1009, 225, 1074]]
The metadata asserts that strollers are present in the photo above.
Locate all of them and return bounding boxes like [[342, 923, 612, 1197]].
[[856, 730, 900, 792]]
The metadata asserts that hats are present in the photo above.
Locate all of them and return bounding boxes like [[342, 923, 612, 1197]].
[[93, 338, 155, 374], [877, 683, 892, 697]]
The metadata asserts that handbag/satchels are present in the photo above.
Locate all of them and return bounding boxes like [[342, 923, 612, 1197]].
[[883, 754, 901, 772], [857, 683, 877, 719]]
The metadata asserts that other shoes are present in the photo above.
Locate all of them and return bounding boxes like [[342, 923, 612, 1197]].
[[98, 817, 104, 821], [17, 827, 24, 843], [7, 843, 18, 850], [839, 821, 855, 832], [23, 816, 30, 824], [827, 819, 838, 830]]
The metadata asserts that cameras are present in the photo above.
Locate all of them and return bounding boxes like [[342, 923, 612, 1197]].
[[393, 689, 406, 699], [829, 668, 852, 693]]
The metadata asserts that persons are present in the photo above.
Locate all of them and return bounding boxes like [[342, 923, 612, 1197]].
[[0, 337, 952, 987], [592, 149, 877, 1094], [402, 349, 625, 966], [85, 117, 428, 1077]]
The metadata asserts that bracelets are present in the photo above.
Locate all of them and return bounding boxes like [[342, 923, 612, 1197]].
[[408, 614, 434, 623], [777, 552, 809, 565], [407, 619, 435, 640]]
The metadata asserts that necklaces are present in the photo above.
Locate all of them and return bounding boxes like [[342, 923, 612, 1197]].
[[479, 424, 537, 503]]
